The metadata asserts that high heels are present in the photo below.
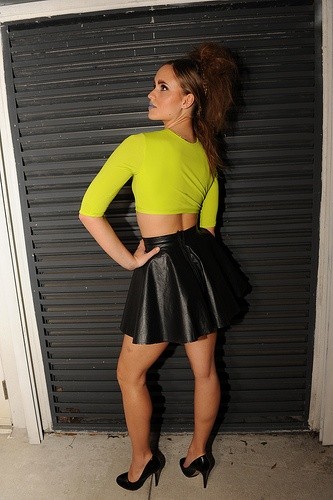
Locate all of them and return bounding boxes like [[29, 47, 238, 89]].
[[116, 454, 166, 492], [180, 456, 213, 488]]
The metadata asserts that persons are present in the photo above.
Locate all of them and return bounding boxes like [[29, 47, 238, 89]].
[[77, 40, 250, 490]]
